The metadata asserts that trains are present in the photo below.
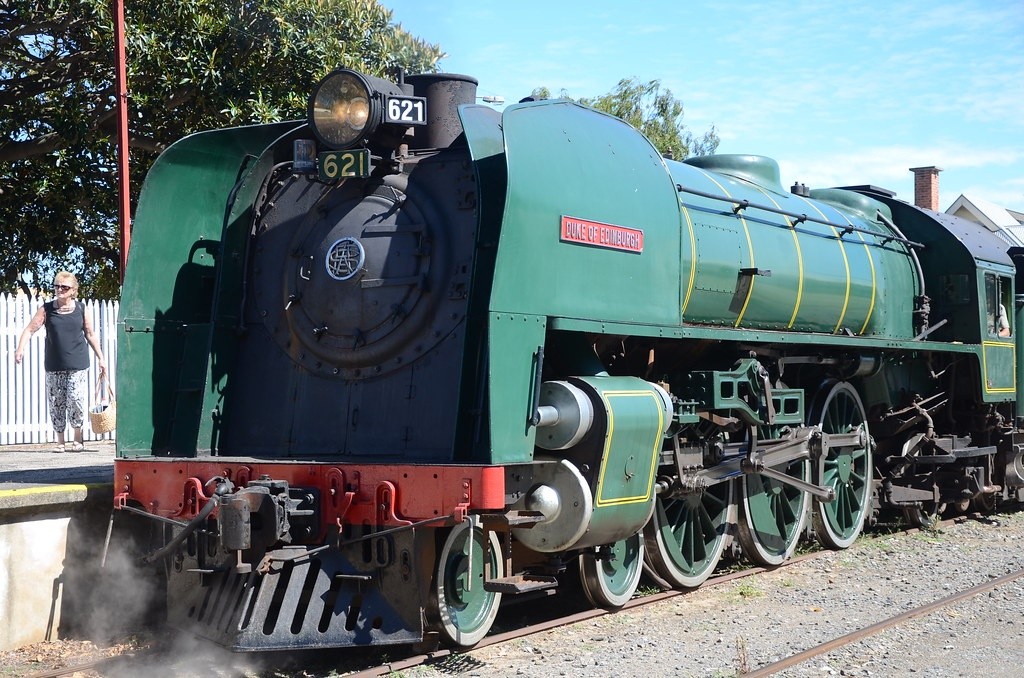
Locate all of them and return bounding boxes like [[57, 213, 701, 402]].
[[78, 66, 1024, 655]]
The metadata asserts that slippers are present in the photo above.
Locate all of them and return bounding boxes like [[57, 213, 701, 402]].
[[72, 441, 85, 451], [52, 444, 66, 452]]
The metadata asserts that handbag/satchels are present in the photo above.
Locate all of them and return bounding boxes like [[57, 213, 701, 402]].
[[88, 370, 117, 435]]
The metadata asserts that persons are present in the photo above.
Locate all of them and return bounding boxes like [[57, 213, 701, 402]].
[[15, 271, 106, 452], [986, 285, 1010, 336]]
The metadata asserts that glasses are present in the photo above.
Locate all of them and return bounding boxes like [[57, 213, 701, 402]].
[[53, 285, 74, 291]]
